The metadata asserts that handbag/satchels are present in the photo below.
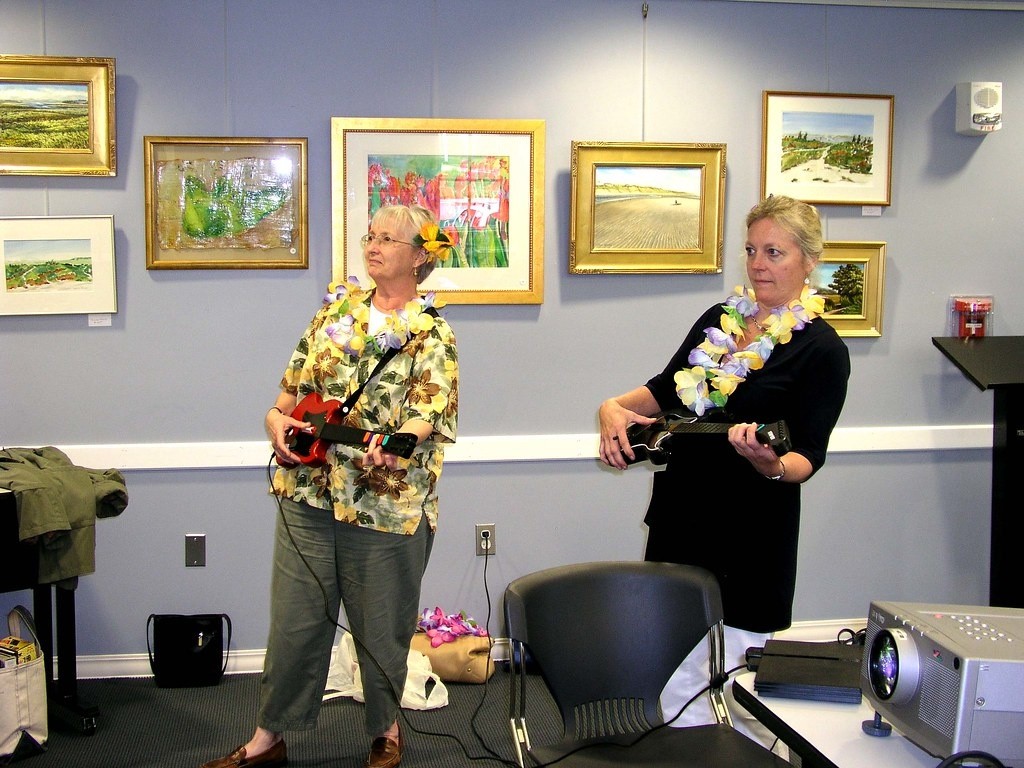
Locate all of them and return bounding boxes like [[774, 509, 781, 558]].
[[146, 613, 232, 688], [410, 632, 496, 685], [0, 605, 48, 756], [322, 630, 448, 711]]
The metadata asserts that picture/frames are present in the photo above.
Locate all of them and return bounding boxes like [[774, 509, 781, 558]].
[[0, 54, 118, 176], [795, 241, 887, 339], [569, 140, 727, 275], [0, 215, 118, 315], [332, 117, 545, 306], [761, 89, 890, 206], [144, 136, 309, 270]]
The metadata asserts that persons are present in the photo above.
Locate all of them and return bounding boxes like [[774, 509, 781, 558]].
[[204, 205, 459, 768], [599, 196, 851, 633]]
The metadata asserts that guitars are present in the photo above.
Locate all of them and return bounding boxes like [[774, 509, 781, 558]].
[[605, 407, 792, 467], [276, 392, 418, 475]]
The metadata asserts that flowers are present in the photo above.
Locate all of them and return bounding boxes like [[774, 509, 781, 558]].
[[325, 277, 447, 356], [416, 607, 488, 648], [413, 220, 456, 264], [674, 281, 826, 417]]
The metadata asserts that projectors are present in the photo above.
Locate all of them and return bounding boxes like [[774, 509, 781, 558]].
[[857, 601, 1024, 768]]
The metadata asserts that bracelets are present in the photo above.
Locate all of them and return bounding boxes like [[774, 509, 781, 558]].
[[765, 461, 785, 481], [268, 407, 284, 415]]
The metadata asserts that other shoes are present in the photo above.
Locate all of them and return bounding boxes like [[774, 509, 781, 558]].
[[366, 720, 403, 768], [201, 738, 288, 768]]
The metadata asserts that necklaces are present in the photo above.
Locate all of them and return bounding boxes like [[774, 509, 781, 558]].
[[674, 284, 824, 416], [324, 274, 447, 355]]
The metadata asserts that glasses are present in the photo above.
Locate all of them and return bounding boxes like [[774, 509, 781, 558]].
[[361, 233, 422, 251]]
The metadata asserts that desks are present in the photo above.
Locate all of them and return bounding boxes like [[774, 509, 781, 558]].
[[1, 487, 91, 720], [932, 337, 1024, 607], [733, 673, 943, 767]]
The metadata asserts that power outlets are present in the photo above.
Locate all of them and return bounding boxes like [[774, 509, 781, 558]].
[[476, 523, 496, 556]]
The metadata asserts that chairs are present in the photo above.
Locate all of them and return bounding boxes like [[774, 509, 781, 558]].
[[504, 560, 792, 768]]
